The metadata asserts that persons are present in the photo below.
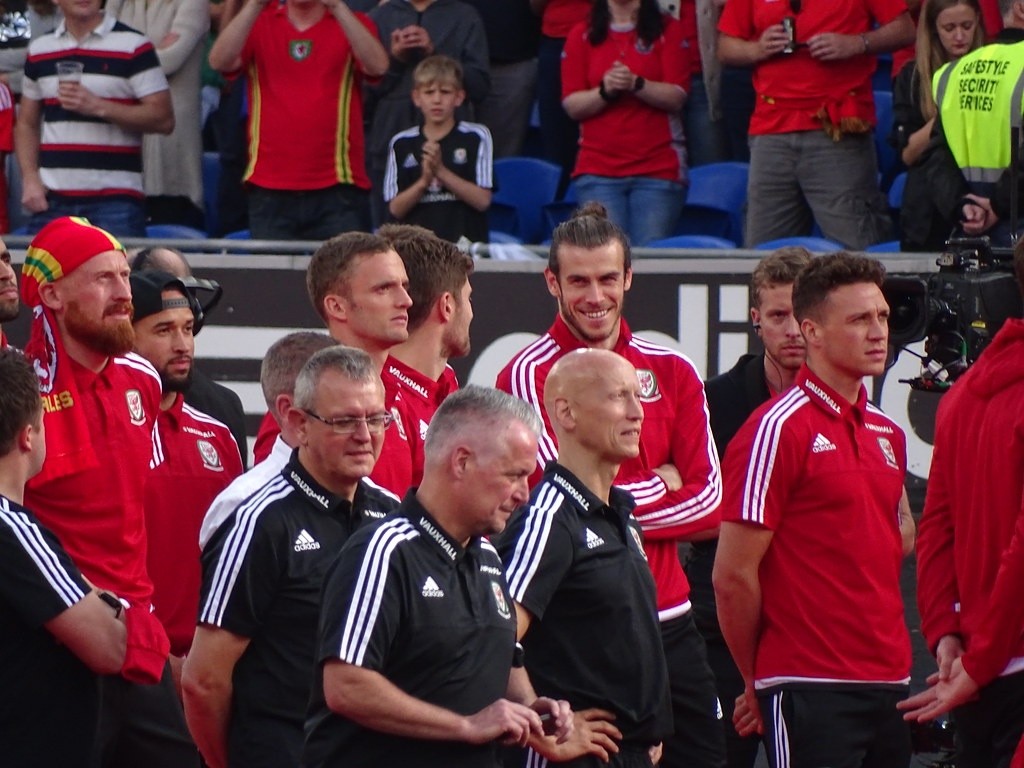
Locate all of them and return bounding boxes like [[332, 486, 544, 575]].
[[380, 51, 496, 245], [11, 214, 209, 768], [493, 202, 728, 768], [182, 346, 404, 767], [313, 388, 575, 768], [1, 0, 1023, 768], [715, 249, 908, 767]]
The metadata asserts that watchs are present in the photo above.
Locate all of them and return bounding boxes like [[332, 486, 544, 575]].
[[630, 75, 645, 94]]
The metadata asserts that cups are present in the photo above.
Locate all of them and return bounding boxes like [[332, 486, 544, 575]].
[[57, 61, 83, 86]]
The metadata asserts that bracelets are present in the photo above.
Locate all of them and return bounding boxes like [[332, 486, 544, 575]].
[[859, 33, 869, 53], [599, 80, 612, 102]]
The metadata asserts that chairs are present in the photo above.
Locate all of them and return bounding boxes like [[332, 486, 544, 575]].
[[5, 50, 911, 251]]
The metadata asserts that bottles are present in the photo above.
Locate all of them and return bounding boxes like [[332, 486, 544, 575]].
[[780, 18, 793, 53]]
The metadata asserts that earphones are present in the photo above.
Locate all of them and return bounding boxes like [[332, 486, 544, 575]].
[[752, 323, 760, 329]]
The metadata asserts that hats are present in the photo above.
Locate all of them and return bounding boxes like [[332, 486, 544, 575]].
[[129, 272, 197, 323]]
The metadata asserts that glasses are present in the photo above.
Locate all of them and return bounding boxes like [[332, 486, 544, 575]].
[[300, 408, 393, 433]]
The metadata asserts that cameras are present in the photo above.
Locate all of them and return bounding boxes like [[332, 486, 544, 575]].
[[779, 17, 808, 54]]
